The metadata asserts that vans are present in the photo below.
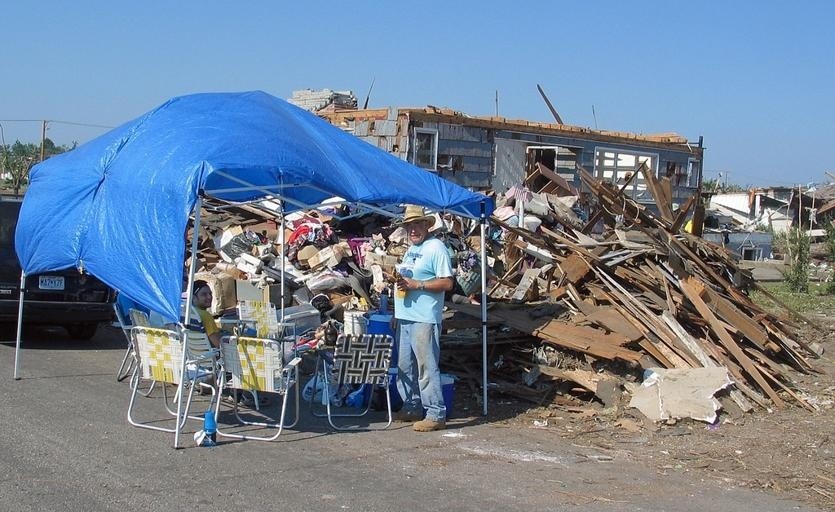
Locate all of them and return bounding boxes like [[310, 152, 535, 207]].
[[1, 197, 118, 340]]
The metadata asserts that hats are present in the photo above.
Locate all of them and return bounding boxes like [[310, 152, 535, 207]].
[[394, 206, 435, 229], [192, 280, 208, 295]]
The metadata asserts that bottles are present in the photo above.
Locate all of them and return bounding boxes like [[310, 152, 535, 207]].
[[325, 321, 338, 346], [203, 406, 216, 445]]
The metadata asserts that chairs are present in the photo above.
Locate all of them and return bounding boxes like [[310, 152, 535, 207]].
[[126, 326, 217, 429], [114, 303, 344, 380], [310, 333, 394, 431], [214, 335, 301, 441]]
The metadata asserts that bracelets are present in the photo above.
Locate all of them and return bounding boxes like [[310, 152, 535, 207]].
[[420, 281, 424, 290]]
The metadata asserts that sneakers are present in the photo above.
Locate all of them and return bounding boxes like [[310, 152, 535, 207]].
[[413, 415, 446, 431], [385, 407, 423, 423], [228, 393, 270, 407]]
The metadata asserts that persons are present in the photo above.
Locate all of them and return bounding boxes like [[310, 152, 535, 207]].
[[386, 204, 455, 433], [190, 279, 271, 407]]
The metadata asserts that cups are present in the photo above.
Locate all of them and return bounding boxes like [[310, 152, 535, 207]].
[[381, 294, 387, 314], [398, 290, 408, 298]]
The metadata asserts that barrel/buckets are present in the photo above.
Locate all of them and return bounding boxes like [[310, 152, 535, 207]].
[[423, 385, 455, 417], [343, 310, 366, 335]]
